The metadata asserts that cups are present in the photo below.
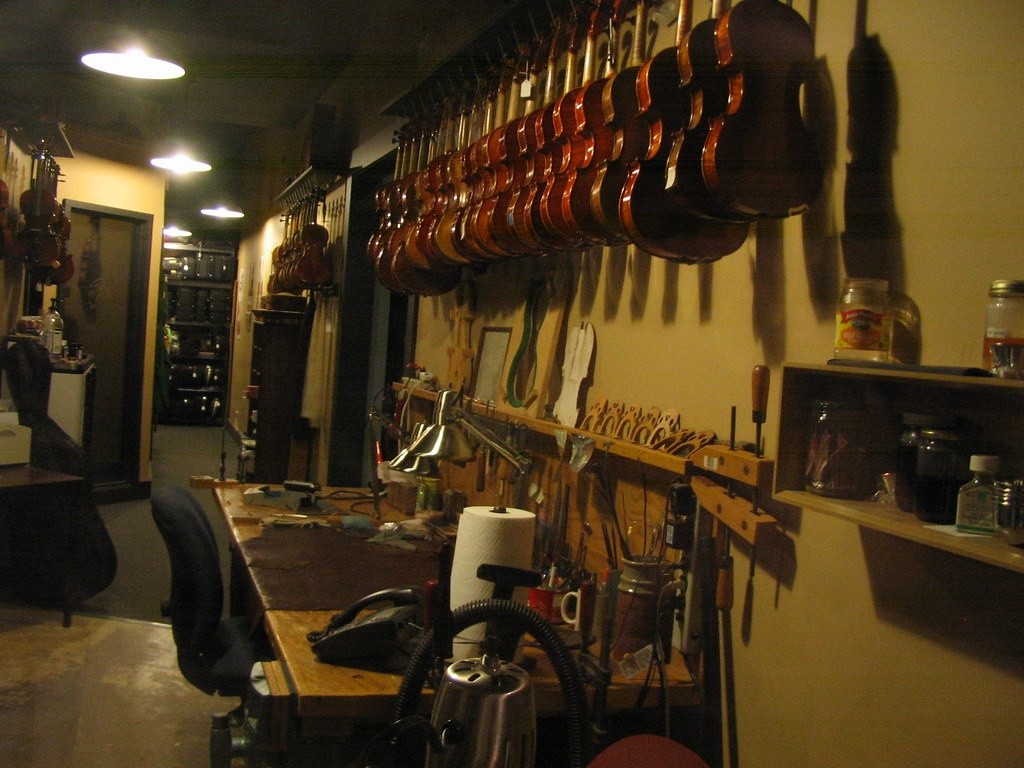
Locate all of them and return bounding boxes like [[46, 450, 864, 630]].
[[990, 478, 1024, 545], [988, 341, 1024, 379], [528, 586, 569, 624], [560, 585, 608, 639]]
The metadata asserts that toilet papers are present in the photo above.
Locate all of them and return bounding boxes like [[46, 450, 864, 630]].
[[446, 506, 536, 642]]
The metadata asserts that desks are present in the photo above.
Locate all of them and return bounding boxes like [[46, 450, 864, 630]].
[[212, 484, 706, 768], [0, 463, 90, 628]]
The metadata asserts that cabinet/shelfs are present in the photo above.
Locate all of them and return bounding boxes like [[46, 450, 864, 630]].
[[48, 354, 97, 451], [237, 310, 312, 483], [151, 245, 237, 426]]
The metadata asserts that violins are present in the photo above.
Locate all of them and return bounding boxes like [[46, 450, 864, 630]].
[[619, 0, 752, 265], [13, 133, 73, 287], [699, 0, 824, 224], [369, 1, 603, 300], [0, 177, 15, 262], [267, 172, 334, 296], [586, 0, 631, 249], [660, 0, 761, 223]]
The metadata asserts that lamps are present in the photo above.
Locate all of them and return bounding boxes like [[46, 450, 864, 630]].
[[164, 227, 193, 237], [81, 0, 186, 80], [150, 78, 213, 183], [201, 197, 245, 219]]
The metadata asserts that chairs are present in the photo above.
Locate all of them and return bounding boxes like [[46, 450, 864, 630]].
[[151, 486, 275, 730]]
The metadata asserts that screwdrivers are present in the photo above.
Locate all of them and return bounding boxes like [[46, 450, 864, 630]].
[[716, 404, 735, 609], [475, 399, 491, 492], [751, 365, 769, 510]]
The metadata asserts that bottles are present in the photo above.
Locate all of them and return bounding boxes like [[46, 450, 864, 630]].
[[412, 476, 441, 516], [981, 279, 1024, 366], [609, 557, 677, 664], [832, 277, 891, 361], [797, 394, 1000, 534], [69, 342, 83, 360]]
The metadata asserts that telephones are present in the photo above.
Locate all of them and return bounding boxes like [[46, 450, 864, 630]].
[[308, 587, 422, 659]]
